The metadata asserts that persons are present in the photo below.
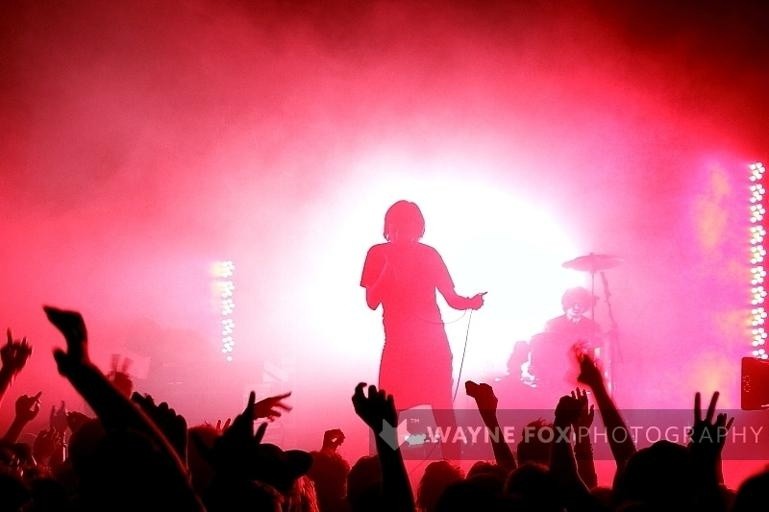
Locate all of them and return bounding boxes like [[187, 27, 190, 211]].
[[0, 200, 769, 512]]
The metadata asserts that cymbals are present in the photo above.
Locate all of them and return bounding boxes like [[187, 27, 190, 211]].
[[563, 254, 623, 270]]
